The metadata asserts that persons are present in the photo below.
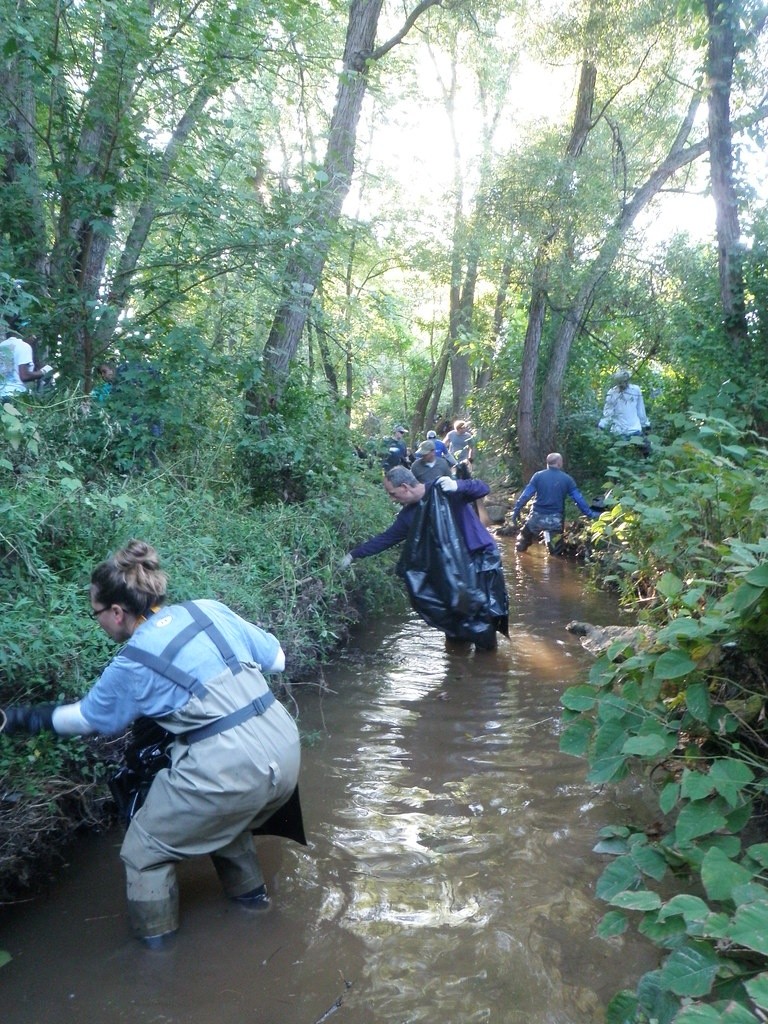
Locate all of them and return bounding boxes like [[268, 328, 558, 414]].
[[597, 370, 652, 460], [377, 425, 411, 474], [512, 453, 603, 559], [337, 465, 513, 655], [411, 440, 452, 486], [0, 540, 308, 955], [412, 430, 458, 469], [0, 318, 55, 411], [441, 420, 475, 463], [96, 337, 167, 470]]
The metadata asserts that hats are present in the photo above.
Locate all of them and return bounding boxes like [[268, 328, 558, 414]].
[[614, 370, 632, 382], [415, 439, 435, 455], [395, 426, 409, 435], [426, 430, 437, 438]]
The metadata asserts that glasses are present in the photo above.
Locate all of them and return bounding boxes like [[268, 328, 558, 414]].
[[88, 605, 130, 621]]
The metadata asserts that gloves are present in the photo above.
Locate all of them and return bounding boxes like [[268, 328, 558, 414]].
[[570, 489, 602, 520], [338, 553, 353, 570], [434, 475, 458, 493], [512, 484, 537, 526], [40, 365, 53, 375], [645, 426, 651, 435], [1, 704, 63, 735]]
